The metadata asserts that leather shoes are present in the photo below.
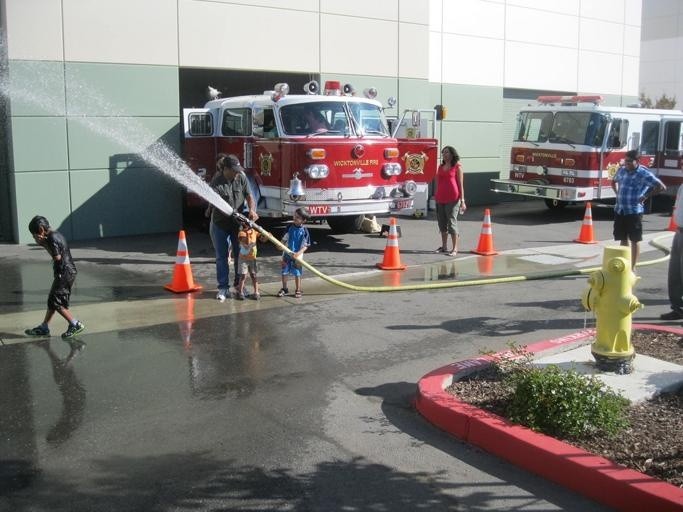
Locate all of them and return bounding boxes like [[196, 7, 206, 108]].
[[661, 310, 680, 320]]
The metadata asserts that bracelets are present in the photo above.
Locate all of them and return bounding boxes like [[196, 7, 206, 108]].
[[459, 198, 466, 203]]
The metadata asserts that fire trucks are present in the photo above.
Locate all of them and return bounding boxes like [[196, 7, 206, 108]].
[[489, 94, 683, 218], [180, 80, 437, 246]]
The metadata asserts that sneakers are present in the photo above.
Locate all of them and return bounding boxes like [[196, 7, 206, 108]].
[[448, 250, 457, 255], [434, 247, 447, 253], [215, 284, 261, 301], [62, 321, 85, 337], [25, 326, 49, 337]]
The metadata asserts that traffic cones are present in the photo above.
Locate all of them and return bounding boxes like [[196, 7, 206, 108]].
[[164, 230, 203, 293], [573, 203, 599, 244], [166, 289, 201, 350], [664, 206, 678, 232], [472, 253, 497, 277], [377, 269, 405, 287], [470, 208, 499, 256], [375, 217, 406, 271]]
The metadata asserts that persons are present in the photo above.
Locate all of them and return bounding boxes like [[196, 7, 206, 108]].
[[25, 215, 85, 339], [433, 145, 467, 256], [661, 155, 683, 319], [612, 150, 667, 272], [207, 105, 347, 300]]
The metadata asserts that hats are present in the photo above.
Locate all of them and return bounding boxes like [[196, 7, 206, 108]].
[[224, 155, 245, 172]]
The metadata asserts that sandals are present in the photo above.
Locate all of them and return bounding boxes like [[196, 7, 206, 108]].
[[277, 288, 287, 296], [295, 290, 302, 298]]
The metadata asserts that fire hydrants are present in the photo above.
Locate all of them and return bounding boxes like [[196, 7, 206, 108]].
[[581, 244, 645, 373]]
